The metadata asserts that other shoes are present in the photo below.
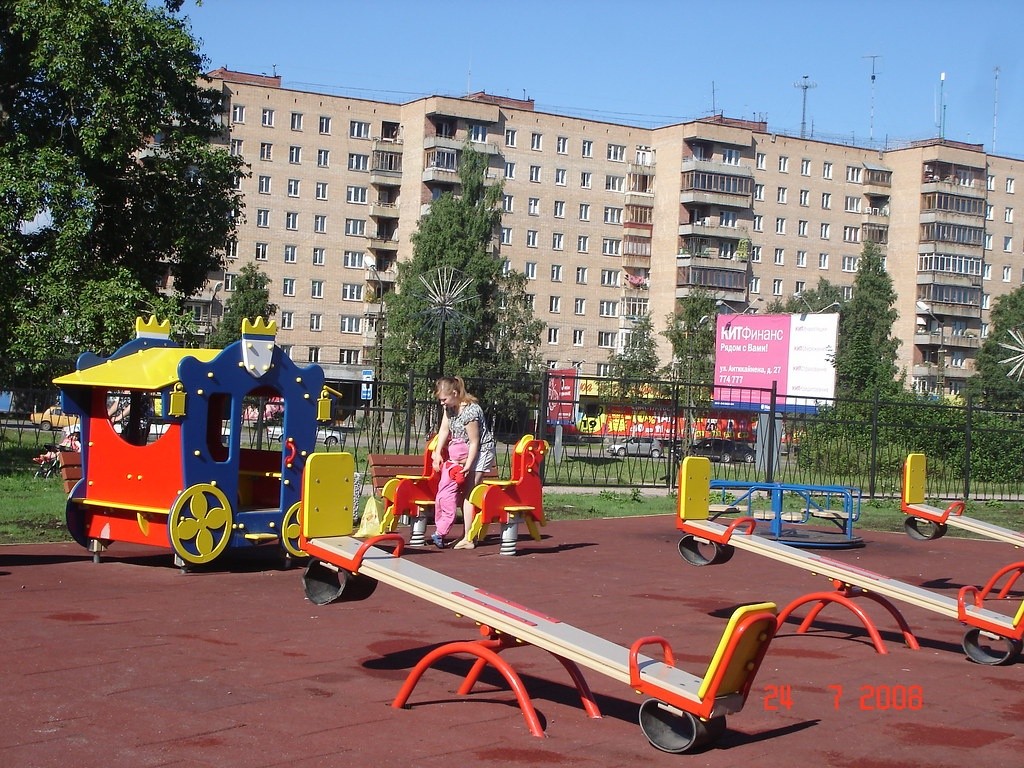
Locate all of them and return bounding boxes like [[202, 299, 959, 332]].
[[430, 534, 444, 548], [33, 458, 43, 464], [40, 455, 49, 462], [448, 538, 474, 549]]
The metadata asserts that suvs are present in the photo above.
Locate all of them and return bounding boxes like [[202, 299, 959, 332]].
[[607, 436, 663, 457]]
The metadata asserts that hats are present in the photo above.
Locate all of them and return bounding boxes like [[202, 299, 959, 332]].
[[66, 426, 80, 437], [447, 439, 469, 461]]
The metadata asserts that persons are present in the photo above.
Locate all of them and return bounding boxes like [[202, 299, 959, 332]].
[[431, 374, 496, 550], [430, 436, 471, 549], [107, 388, 150, 446], [33, 426, 84, 465]]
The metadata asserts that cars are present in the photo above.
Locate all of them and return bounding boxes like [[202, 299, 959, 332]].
[[62, 419, 230, 448], [690, 438, 758, 462], [31, 407, 80, 430], [267, 424, 346, 446]]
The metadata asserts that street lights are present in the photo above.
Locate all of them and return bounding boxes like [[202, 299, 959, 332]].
[[794, 292, 838, 312], [204, 283, 223, 346], [916, 299, 945, 404], [364, 256, 383, 455], [715, 297, 763, 312], [687, 316, 710, 458]]
